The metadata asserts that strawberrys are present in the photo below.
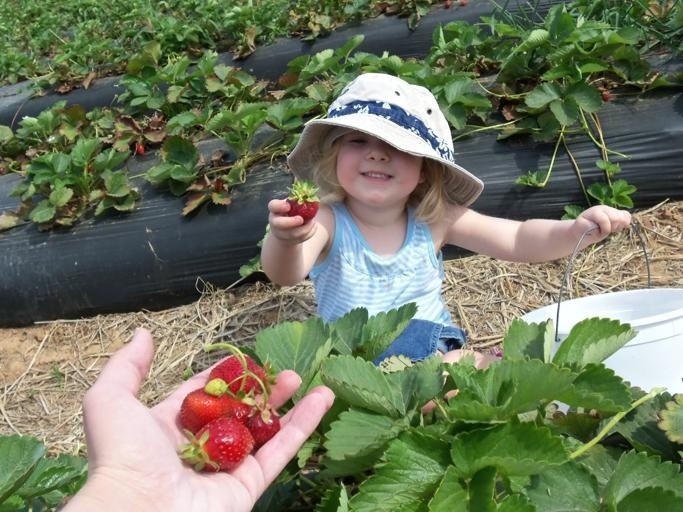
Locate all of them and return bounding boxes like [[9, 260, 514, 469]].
[[286, 180, 320, 223], [177, 343, 280, 473]]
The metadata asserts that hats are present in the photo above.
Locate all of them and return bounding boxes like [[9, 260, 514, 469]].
[[287, 71, 484, 210]]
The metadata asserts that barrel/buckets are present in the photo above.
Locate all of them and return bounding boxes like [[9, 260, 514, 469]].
[[519, 224, 683, 393]]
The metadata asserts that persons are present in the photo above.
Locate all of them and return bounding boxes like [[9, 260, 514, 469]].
[[58, 327, 335, 512], [261, 73, 633, 417]]
